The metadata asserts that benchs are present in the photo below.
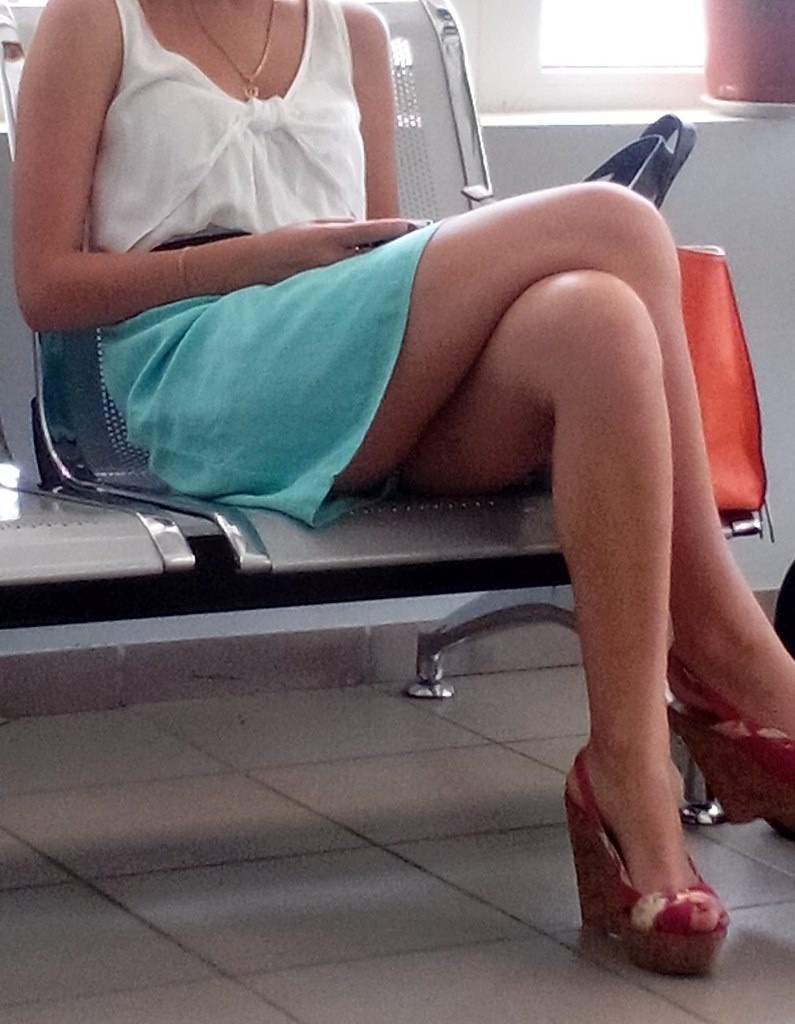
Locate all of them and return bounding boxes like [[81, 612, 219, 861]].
[[0, 0, 763, 828]]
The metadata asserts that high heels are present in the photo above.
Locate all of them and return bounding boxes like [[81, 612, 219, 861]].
[[667, 642, 795, 823], [564, 746, 729, 976]]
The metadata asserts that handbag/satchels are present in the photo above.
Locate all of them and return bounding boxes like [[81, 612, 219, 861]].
[[582, 112, 767, 511]]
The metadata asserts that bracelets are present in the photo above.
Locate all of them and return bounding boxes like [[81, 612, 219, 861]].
[[177, 244, 192, 301]]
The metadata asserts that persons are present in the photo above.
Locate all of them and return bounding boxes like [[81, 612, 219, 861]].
[[12, 0, 795, 973]]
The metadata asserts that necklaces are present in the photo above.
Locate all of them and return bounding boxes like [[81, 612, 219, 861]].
[[189, 0, 278, 100]]
